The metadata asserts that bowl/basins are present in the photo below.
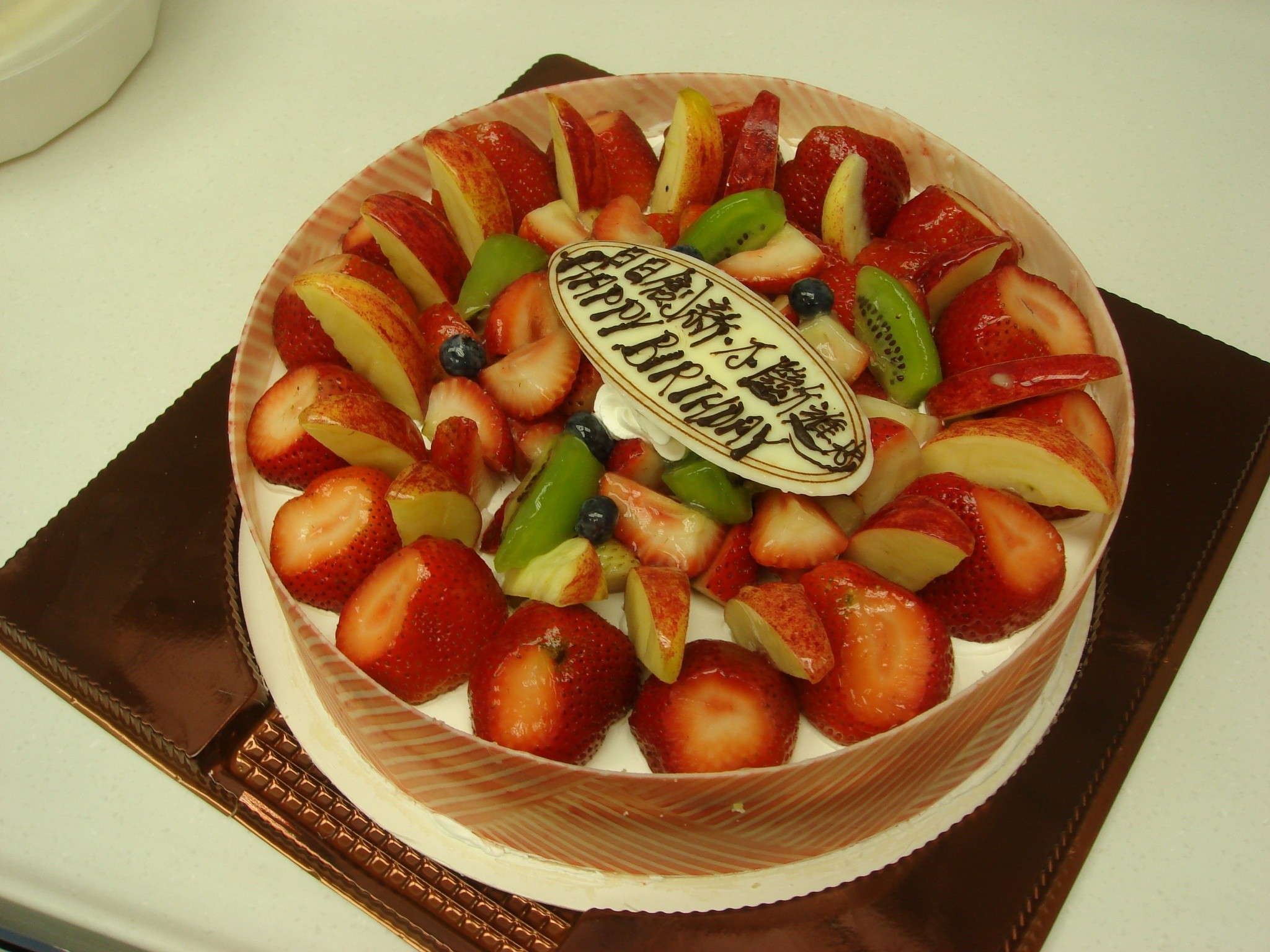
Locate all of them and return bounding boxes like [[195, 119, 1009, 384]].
[[0, 0, 164, 166]]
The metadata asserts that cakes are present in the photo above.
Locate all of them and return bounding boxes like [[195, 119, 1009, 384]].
[[225, 70, 1133, 876]]
